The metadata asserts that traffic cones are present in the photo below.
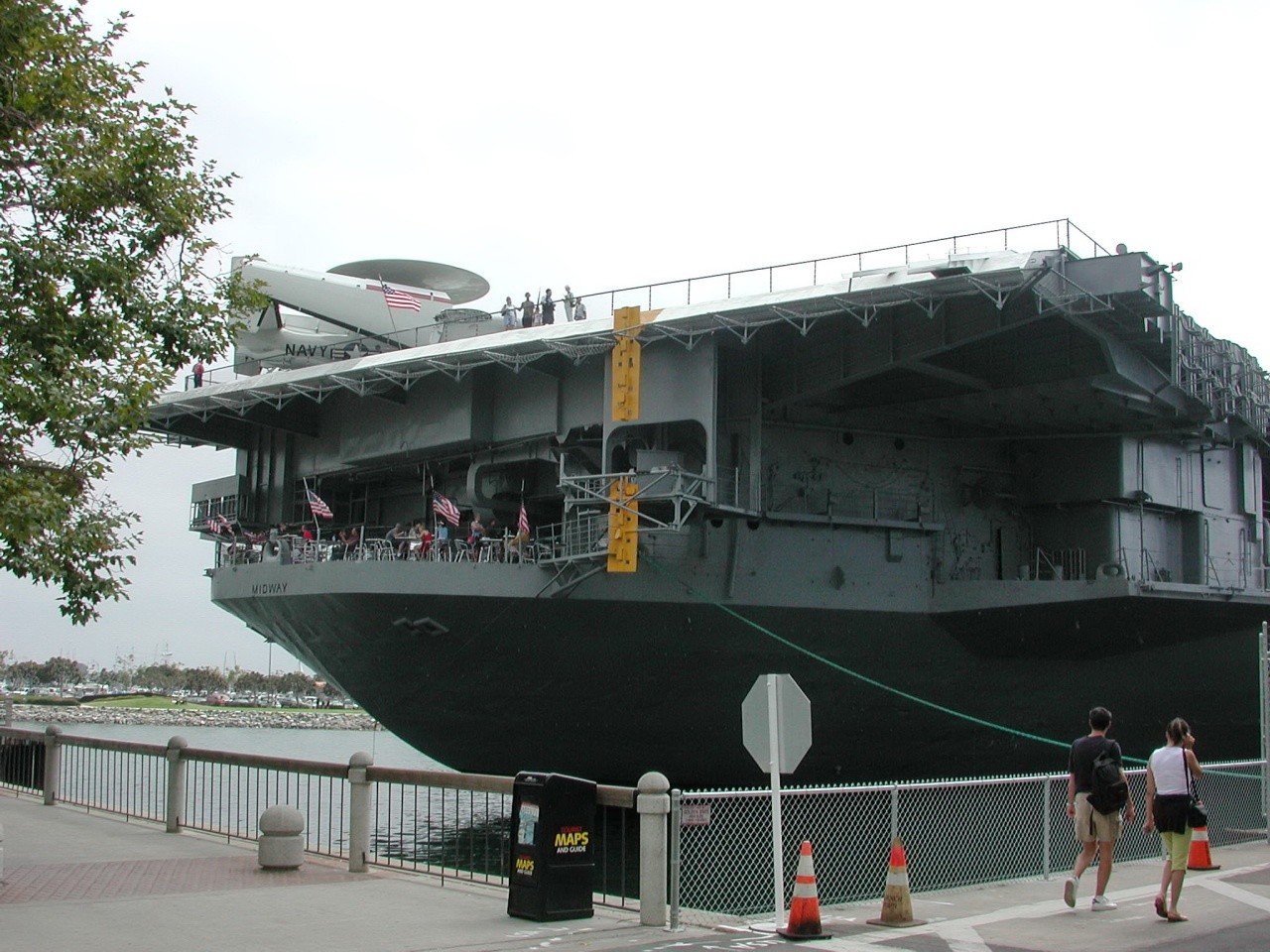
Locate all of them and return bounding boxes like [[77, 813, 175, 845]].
[[776, 840, 834, 941], [865, 838, 929, 929], [1182, 799, 1222, 871]]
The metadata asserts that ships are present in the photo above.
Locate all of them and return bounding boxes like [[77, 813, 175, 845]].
[[94, 219, 1269, 899]]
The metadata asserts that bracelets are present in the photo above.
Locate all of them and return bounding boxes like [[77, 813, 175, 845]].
[[1067, 803, 1074, 806]]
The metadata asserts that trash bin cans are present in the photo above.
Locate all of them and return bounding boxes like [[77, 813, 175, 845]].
[[507, 771, 598, 920]]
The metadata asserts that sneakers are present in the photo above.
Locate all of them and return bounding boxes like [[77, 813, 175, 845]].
[[1091, 895, 1117, 910], [1064, 878, 1077, 908]]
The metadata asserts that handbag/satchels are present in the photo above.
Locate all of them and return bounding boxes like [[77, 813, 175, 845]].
[[1187, 803, 1208, 828]]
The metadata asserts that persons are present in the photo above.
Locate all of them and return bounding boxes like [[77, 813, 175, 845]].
[[301, 522, 359, 551], [1064, 707, 1133, 911], [1142, 717, 1202, 921], [502, 285, 586, 328], [469, 513, 502, 562], [385, 521, 448, 562], [192, 358, 204, 388]]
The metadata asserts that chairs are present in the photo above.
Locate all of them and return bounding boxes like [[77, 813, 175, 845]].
[[228, 539, 537, 565]]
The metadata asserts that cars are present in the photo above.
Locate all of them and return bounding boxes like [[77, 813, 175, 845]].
[[0, 677, 160, 700], [170, 689, 360, 710]]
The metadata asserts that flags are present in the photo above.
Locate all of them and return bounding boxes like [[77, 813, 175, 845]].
[[515, 507, 532, 540], [383, 284, 421, 314], [308, 490, 334, 517], [432, 490, 461, 527]]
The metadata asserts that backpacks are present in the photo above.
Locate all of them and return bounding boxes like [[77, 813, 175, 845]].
[[1085, 740, 1128, 815]]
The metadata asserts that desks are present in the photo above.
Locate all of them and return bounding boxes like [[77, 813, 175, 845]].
[[277, 535, 299, 563], [478, 539, 502, 563], [309, 540, 335, 563], [396, 537, 419, 562]]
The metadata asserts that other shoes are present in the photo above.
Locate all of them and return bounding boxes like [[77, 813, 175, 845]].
[[1167, 911, 1188, 922], [1155, 894, 1168, 918]]
